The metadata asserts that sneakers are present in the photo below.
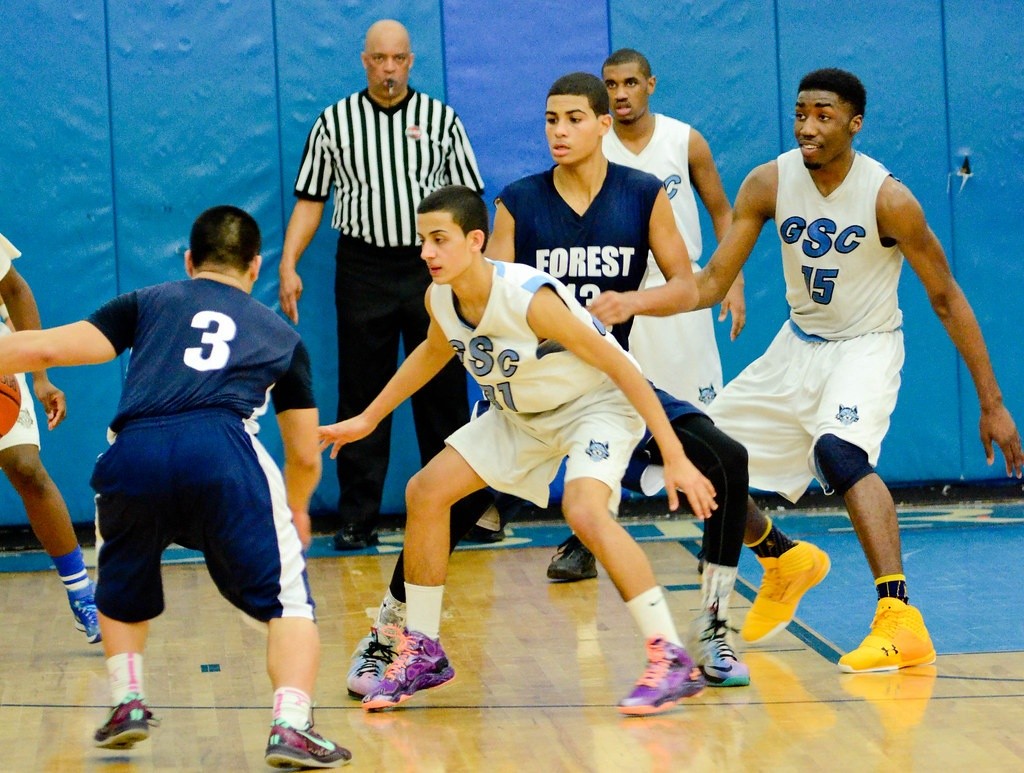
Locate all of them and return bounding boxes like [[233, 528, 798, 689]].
[[66, 578, 102, 644], [546, 579, 599, 623], [464, 525, 506, 543], [838, 597, 936, 673], [840, 664, 937, 727], [547, 533, 599, 579], [334, 529, 380, 551], [265, 718, 352, 768], [93, 699, 161, 749], [345, 627, 400, 700], [742, 539, 831, 643], [618, 638, 706, 715], [695, 598, 751, 687], [362, 627, 455, 711]]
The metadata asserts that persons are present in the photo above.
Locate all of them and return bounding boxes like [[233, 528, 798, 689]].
[[0, 206, 351, 770], [546, 48, 745, 581], [0, 234, 104, 645], [316, 185, 707, 714], [347, 72, 750, 699], [279, 20, 505, 552], [624, 68, 1024, 674]]
